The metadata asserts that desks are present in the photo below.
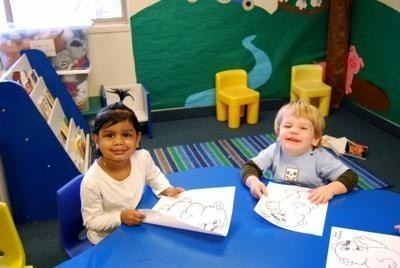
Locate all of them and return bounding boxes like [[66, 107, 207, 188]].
[[49, 165, 400, 268]]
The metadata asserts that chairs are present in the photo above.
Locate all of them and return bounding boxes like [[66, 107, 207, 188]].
[[56, 174, 93, 258], [215, 69, 260, 129], [99, 80, 154, 139], [0, 202, 33, 268], [290, 63, 332, 117]]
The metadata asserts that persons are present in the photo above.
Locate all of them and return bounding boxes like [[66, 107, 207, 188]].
[[79, 88, 185, 247], [239, 100, 358, 205]]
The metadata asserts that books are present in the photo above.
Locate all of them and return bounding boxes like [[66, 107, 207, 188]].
[[319, 134, 337, 150], [344, 140, 369, 160], [326, 136, 356, 158], [6, 65, 86, 174]]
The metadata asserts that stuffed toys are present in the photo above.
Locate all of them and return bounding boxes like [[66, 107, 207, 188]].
[[0, 29, 89, 71]]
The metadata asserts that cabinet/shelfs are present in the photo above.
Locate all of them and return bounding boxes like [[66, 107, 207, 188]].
[[55, 66, 103, 117], [0, 49, 92, 223]]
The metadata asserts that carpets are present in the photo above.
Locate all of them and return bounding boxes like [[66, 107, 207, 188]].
[[147, 133, 393, 189]]
